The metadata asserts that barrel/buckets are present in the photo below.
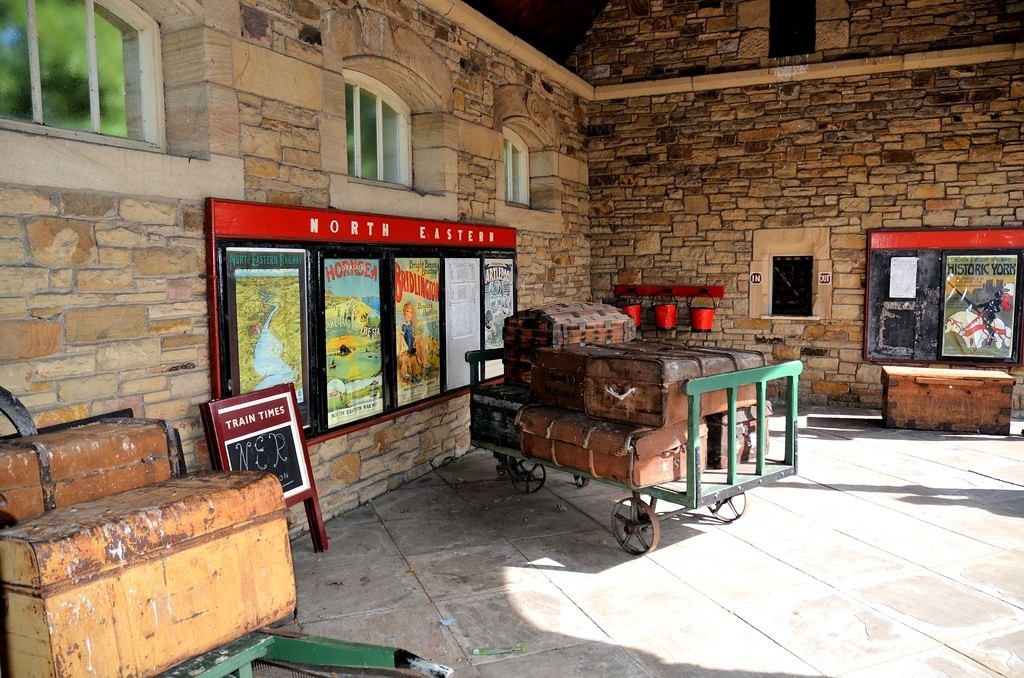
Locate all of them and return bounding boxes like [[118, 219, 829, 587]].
[[650, 288, 678, 331], [688, 291, 717, 333], [616, 288, 643, 330]]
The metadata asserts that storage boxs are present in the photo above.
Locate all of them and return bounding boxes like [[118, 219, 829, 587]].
[[467, 298, 773, 490], [881, 365, 1016, 436], [0, 418, 298, 678]]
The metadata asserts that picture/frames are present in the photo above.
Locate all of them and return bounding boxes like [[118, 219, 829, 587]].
[[936, 246, 1024, 366], [319, 241, 395, 435], [442, 244, 483, 397], [390, 243, 445, 412], [214, 238, 318, 442], [481, 247, 518, 385]]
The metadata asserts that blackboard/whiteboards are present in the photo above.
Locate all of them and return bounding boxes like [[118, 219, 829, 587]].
[[199, 382, 316, 507]]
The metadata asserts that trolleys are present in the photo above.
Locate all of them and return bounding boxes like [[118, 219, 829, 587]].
[[463, 344, 805, 556], [0, 383, 453, 677]]
